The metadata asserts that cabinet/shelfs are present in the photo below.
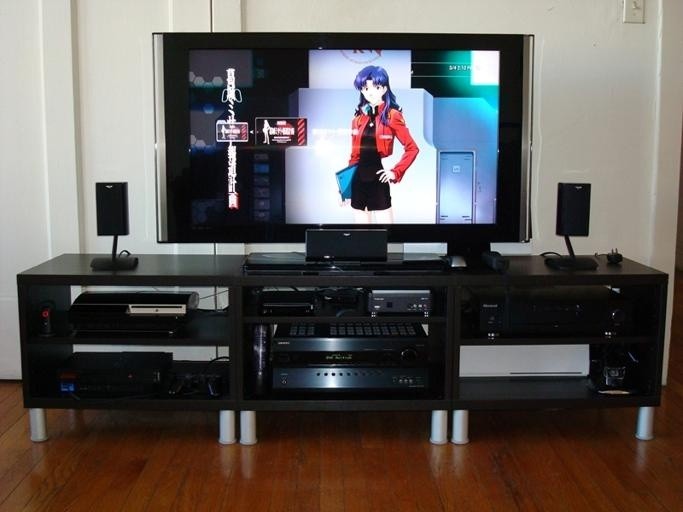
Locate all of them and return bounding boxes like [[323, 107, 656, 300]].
[[447, 255, 668, 443], [15, 254, 246, 446], [236, 255, 455, 446]]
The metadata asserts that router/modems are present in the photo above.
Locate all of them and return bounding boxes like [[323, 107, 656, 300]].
[[545, 254, 598, 271]]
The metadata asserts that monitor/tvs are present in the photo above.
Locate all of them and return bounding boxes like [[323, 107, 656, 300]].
[[556, 182, 591, 236], [152, 31, 535, 274]]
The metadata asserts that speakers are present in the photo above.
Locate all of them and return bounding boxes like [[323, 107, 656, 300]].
[[96, 181, 129, 235]]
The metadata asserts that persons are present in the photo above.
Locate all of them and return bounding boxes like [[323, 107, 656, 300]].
[[348, 65, 420, 222]]
